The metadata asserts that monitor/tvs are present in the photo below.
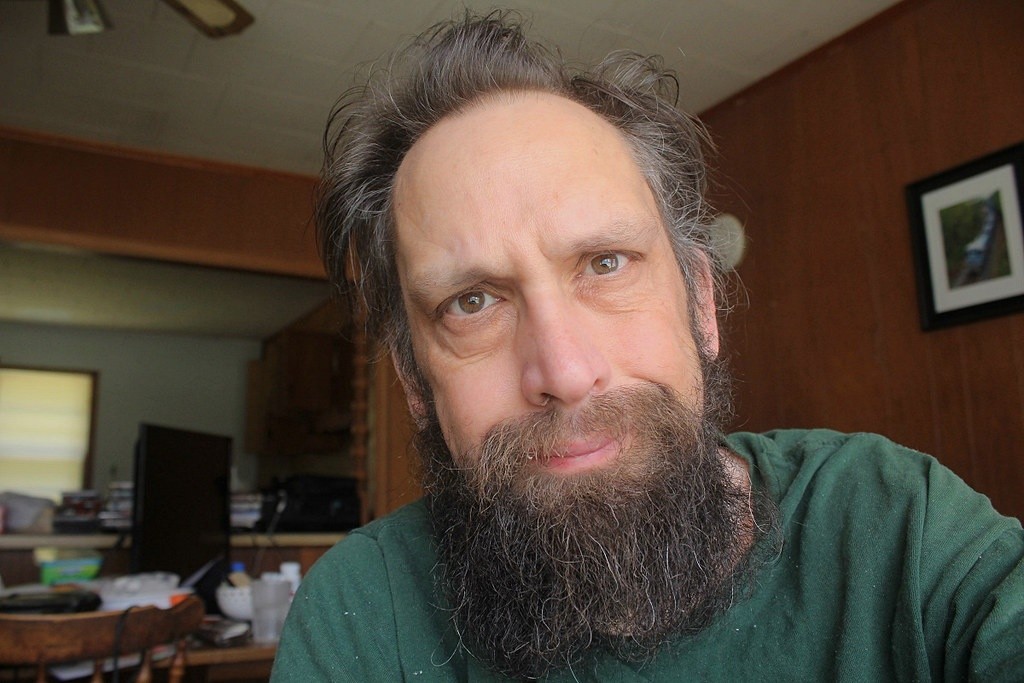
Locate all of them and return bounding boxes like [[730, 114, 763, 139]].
[[129, 421, 232, 612]]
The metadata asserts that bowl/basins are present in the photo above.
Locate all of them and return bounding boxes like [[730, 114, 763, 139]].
[[218, 585, 251, 622]]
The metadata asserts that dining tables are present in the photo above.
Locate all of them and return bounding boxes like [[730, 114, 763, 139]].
[[0, 584, 276, 683]]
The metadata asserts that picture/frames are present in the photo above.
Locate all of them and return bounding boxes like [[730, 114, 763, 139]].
[[906, 141, 1024, 331]]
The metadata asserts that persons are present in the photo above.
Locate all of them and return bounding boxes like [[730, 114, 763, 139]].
[[268, 7, 1021, 682]]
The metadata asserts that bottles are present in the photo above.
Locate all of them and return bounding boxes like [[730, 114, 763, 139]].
[[228, 562, 253, 586]]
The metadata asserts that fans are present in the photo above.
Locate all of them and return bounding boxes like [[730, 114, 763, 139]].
[[47, 0, 254, 40]]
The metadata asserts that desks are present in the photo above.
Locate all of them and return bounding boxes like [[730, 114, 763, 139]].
[[0, 532, 348, 590]]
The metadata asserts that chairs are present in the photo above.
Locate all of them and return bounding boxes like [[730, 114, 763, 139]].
[[0, 596, 205, 683]]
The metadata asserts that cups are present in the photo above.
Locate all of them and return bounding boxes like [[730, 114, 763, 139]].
[[250, 579, 291, 644]]
